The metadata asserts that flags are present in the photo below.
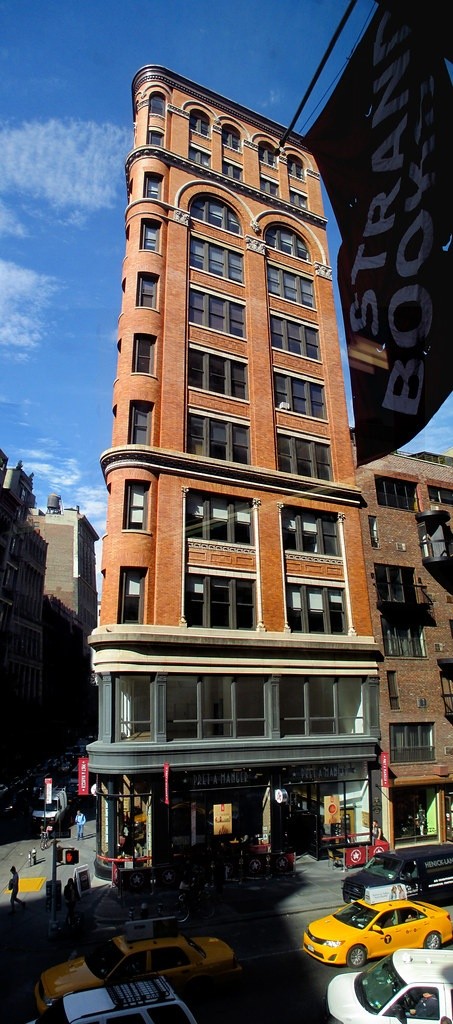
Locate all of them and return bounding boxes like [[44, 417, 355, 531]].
[[301, 2, 453, 470]]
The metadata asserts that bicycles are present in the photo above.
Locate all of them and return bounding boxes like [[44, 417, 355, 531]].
[[40, 833, 52, 850]]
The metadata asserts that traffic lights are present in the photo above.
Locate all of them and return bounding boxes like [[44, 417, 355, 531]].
[[65, 850, 80, 865]]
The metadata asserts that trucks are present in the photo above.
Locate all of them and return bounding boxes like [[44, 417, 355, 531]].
[[33, 786, 67, 826]]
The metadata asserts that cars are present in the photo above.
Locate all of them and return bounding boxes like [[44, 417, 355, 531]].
[[32, 934, 242, 1015], [302, 885, 452, 969]]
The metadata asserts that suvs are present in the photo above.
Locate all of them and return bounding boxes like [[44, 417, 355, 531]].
[[27, 972, 196, 1024], [326, 948, 453, 1023], [343, 843, 453, 908]]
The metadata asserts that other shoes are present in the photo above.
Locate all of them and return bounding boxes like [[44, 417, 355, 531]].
[[77, 838, 79, 841], [80, 838, 83, 840], [8, 911, 16, 915], [22, 902, 26, 910]]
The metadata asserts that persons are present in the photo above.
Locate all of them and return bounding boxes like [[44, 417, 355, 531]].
[[371, 819, 387, 842], [75, 810, 86, 840], [390, 885, 405, 900], [415, 804, 426, 836], [404, 914, 417, 922], [64, 880, 80, 925], [9, 866, 26, 914], [377, 913, 393, 928]]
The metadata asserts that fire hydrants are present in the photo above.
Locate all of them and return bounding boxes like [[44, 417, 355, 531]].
[[32, 848, 38, 866]]
